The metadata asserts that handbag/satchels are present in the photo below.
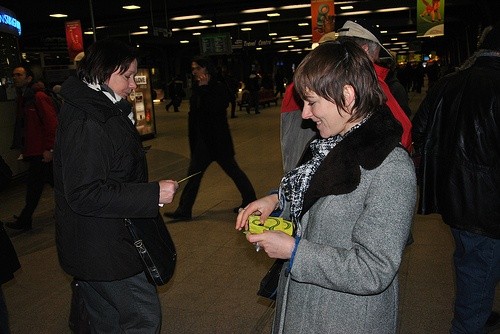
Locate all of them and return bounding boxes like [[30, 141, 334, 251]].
[[123, 215, 179, 285]]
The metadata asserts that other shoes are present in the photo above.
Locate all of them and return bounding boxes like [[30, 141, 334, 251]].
[[164, 211, 194, 221], [234, 204, 246, 213], [5, 214, 34, 229]]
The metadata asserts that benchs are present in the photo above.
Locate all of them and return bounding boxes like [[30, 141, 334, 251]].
[[237, 88, 280, 112]]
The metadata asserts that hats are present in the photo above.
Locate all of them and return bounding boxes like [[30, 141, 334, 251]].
[[337, 17, 394, 62]]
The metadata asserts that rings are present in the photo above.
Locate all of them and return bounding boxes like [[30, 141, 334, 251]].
[[255, 242, 259, 252], [238, 208, 244, 212]]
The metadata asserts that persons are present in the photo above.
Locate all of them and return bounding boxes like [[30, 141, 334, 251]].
[[53, 38, 179, 334], [411, 25, 500, 334], [235, 35, 417, 334], [163, 20, 456, 301], [5, 67, 59, 231]]
